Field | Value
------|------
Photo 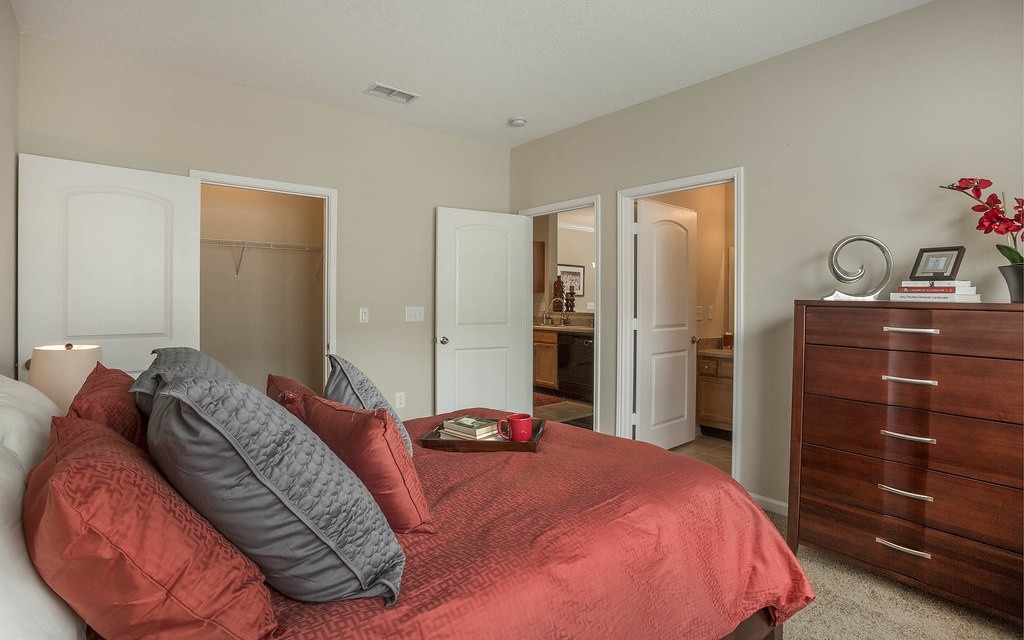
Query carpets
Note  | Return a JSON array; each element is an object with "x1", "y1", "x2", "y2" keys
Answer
[
  {"x1": 762, "y1": 509, "x2": 1024, "y2": 640},
  {"x1": 533, "y1": 392, "x2": 565, "y2": 407}
]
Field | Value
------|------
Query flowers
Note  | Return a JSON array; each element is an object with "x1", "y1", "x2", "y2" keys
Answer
[{"x1": 939, "y1": 178, "x2": 1024, "y2": 264}]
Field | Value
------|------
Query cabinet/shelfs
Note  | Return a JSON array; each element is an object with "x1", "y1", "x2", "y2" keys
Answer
[
  {"x1": 696, "y1": 355, "x2": 734, "y2": 432},
  {"x1": 786, "y1": 300, "x2": 1024, "y2": 628},
  {"x1": 533, "y1": 329, "x2": 570, "y2": 392}
]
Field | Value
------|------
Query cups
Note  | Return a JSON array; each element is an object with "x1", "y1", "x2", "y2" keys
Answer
[{"x1": 497, "y1": 413, "x2": 532, "y2": 442}]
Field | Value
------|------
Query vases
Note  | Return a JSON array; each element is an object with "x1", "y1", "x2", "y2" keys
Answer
[{"x1": 998, "y1": 265, "x2": 1024, "y2": 303}]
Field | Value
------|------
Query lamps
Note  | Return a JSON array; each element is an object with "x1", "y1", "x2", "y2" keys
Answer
[{"x1": 27, "y1": 343, "x2": 103, "y2": 415}]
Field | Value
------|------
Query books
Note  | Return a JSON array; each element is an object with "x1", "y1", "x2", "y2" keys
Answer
[
  {"x1": 437, "y1": 414, "x2": 506, "y2": 443},
  {"x1": 889, "y1": 280, "x2": 981, "y2": 303}
]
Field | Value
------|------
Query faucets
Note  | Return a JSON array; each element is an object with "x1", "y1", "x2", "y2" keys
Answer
[{"x1": 548, "y1": 298, "x2": 565, "y2": 318}]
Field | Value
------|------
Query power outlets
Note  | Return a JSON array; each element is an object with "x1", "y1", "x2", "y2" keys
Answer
[{"x1": 395, "y1": 392, "x2": 406, "y2": 408}]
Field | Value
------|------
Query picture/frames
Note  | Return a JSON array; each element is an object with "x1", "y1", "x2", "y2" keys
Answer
[
  {"x1": 557, "y1": 264, "x2": 585, "y2": 297},
  {"x1": 909, "y1": 246, "x2": 966, "y2": 280}
]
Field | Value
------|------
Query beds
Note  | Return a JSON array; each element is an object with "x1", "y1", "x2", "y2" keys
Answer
[{"x1": 1, "y1": 374, "x2": 815, "y2": 639}]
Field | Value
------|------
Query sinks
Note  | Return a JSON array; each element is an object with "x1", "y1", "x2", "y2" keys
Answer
[{"x1": 533, "y1": 323, "x2": 559, "y2": 330}]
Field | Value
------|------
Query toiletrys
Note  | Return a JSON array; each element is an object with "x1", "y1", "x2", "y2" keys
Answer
[
  {"x1": 546, "y1": 312, "x2": 552, "y2": 325},
  {"x1": 542, "y1": 310, "x2": 547, "y2": 325}
]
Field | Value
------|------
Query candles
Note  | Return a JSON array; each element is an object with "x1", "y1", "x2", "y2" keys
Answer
[
  {"x1": 570, "y1": 286, "x2": 574, "y2": 293},
  {"x1": 566, "y1": 292, "x2": 570, "y2": 299}
]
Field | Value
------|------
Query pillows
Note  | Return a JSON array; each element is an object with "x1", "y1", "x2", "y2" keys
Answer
[
  {"x1": 129, "y1": 347, "x2": 239, "y2": 417},
  {"x1": 23, "y1": 416, "x2": 279, "y2": 639},
  {"x1": 147, "y1": 373, "x2": 405, "y2": 607},
  {"x1": 324, "y1": 354, "x2": 413, "y2": 458},
  {"x1": 66, "y1": 361, "x2": 148, "y2": 454},
  {"x1": 267, "y1": 373, "x2": 437, "y2": 535}
]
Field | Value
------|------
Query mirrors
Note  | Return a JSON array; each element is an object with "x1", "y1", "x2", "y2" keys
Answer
[{"x1": 548, "y1": 207, "x2": 595, "y2": 315}]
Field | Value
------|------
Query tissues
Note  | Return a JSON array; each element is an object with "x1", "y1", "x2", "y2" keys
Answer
[{"x1": 722, "y1": 331, "x2": 734, "y2": 349}]
